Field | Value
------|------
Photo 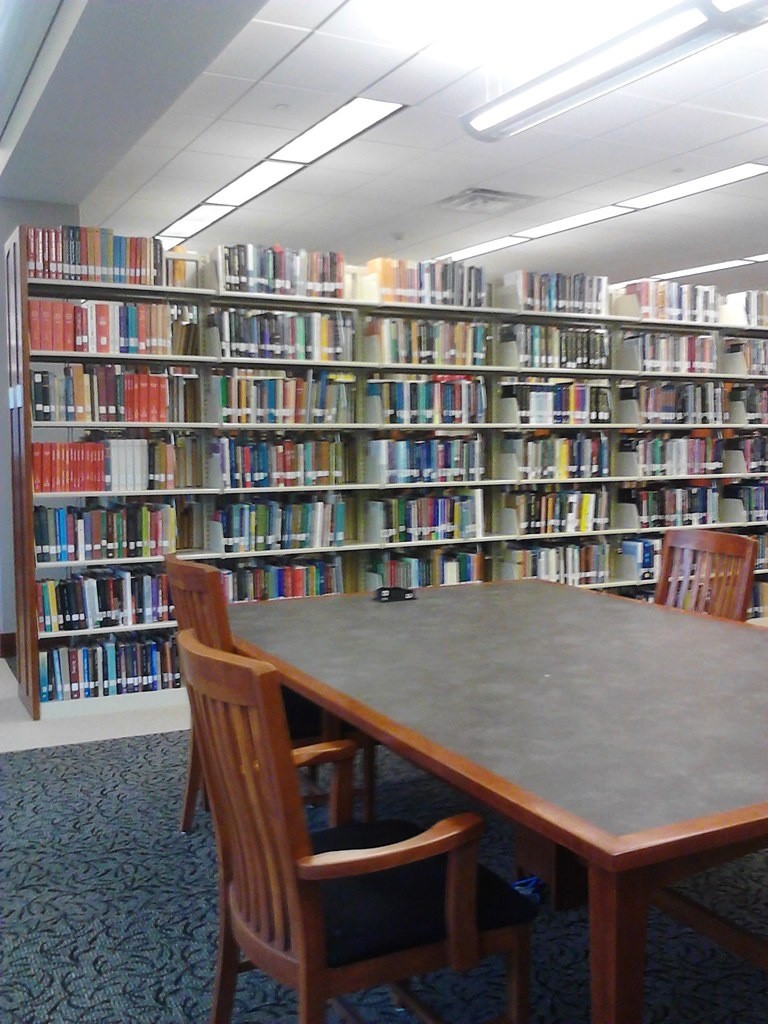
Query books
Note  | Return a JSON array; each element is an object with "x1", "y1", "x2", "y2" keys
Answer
[{"x1": 21, "y1": 222, "x2": 768, "y2": 705}]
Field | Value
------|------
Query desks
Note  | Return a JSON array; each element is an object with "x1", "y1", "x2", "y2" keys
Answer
[{"x1": 209, "y1": 575, "x2": 768, "y2": 1024}]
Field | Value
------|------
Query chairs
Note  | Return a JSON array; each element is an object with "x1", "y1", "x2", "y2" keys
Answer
[
  {"x1": 171, "y1": 627, "x2": 536, "y2": 1024},
  {"x1": 654, "y1": 530, "x2": 759, "y2": 624},
  {"x1": 162, "y1": 553, "x2": 376, "y2": 834}
]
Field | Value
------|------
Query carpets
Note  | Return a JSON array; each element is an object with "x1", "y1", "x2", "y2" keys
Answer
[{"x1": 0, "y1": 727, "x2": 767, "y2": 1024}]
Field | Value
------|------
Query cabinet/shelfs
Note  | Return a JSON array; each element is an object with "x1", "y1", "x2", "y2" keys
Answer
[{"x1": 1, "y1": 224, "x2": 768, "y2": 721}]
